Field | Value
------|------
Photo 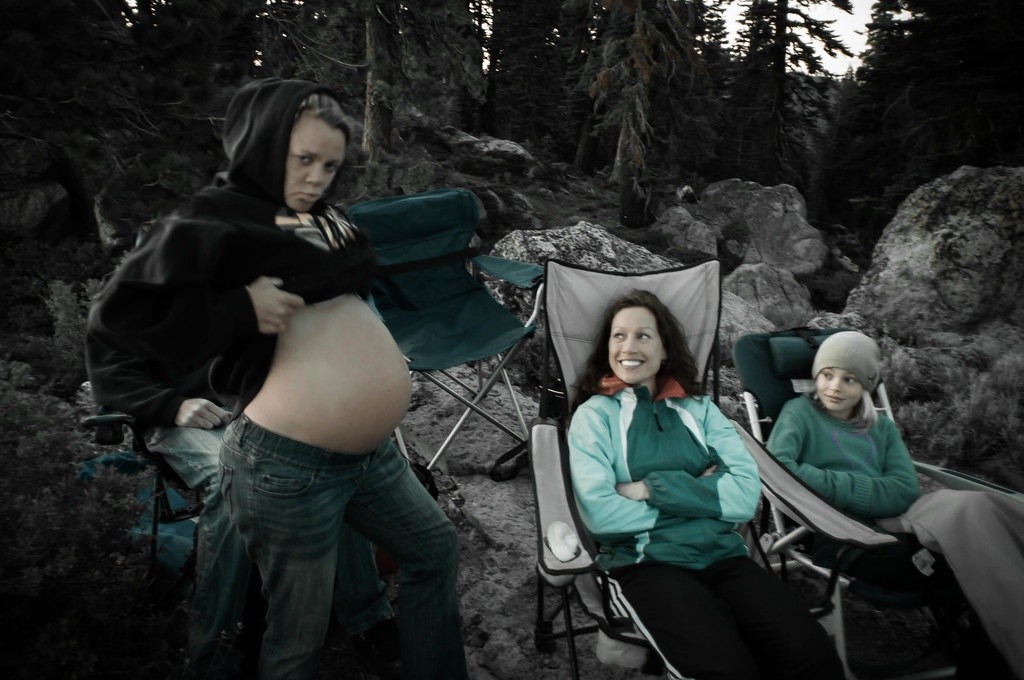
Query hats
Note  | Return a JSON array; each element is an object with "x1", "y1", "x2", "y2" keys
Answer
[{"x1": 812, "y1": 331, "x2": 880, "y2": 391}]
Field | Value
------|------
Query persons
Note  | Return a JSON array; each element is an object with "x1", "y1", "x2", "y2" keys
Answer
[
  {"x1": 79, "y1": 82, "x2": 412, "y2": 679},
  {"x1": 569, "y1": 289, "x2": 848, "y2": 680},
  {"x1": 767, "y1": 325, "x2": 1023, "y2": 679},
  {"x1": 145, "y1": 77, "x2": 467, "y2": 680}
]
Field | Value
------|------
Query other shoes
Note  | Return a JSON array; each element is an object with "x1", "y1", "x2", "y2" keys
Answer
[{"x1": 347, "y1": 617, "x2": 402, "y2": 678}]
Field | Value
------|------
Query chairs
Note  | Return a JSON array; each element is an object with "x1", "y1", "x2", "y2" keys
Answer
[
  {"x1": 82, "y1": 404, "x2": 204, "y2": 580},
  {"x1": 392, "y1": 253, "x2": 545, "y2": 476},
  {"x1": 528, "y1": 258, "x2": 1024, "y2": 680}
]
